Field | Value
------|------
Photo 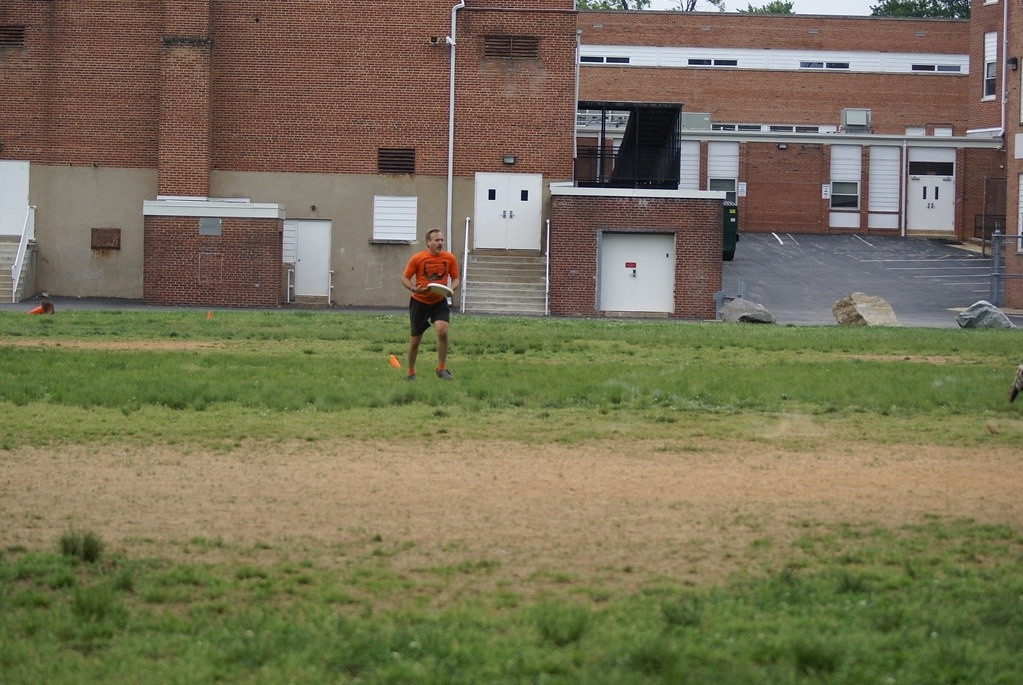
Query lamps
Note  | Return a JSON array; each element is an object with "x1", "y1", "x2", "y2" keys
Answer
[
  {"x1": 777, "y1": 143, "x2": 788, "y2": 149},
  {"x1": 1006, "y1": 57, "x2": 1017, "y2": 70},
  {"x1": 502, "y1": 156, "x2": 516, "y2": 164}
]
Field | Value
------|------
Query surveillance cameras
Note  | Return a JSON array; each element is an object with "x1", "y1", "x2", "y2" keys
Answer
[{"x1": 446, "y1": 36, "x2": 456, "y2": 46}]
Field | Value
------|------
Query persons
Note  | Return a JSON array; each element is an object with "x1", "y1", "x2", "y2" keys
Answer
[{"x1": 402, "y1": 229, "x2": 460, "y2": 382}]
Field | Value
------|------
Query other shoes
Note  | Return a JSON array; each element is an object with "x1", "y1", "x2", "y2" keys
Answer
[
  {"x1": 408, "y1": 374, "x2": 416, "y2": 382},
  {"x1": 436, "y1": 368, "x2": 452, "y2": 380}
]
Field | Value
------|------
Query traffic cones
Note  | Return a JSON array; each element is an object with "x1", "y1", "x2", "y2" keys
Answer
[
  {"x1": 207, "y1": 310, "x2": 212, "y2": 319},
  {"x1": 23, "y1": 302, "x2": 54, "y2": 314},
  {"x1": 389, "y1": 355, "x2": 403, "y2": 370}
]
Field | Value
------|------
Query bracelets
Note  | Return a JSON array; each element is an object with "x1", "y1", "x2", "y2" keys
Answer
[{"x1": 412, "y1": 288, "x2": 414, "y2": 292}]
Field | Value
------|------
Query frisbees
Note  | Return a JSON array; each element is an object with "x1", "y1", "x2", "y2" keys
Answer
[{"x1": 427, "y1": 282, "x2": 452, "y2": 296}]
